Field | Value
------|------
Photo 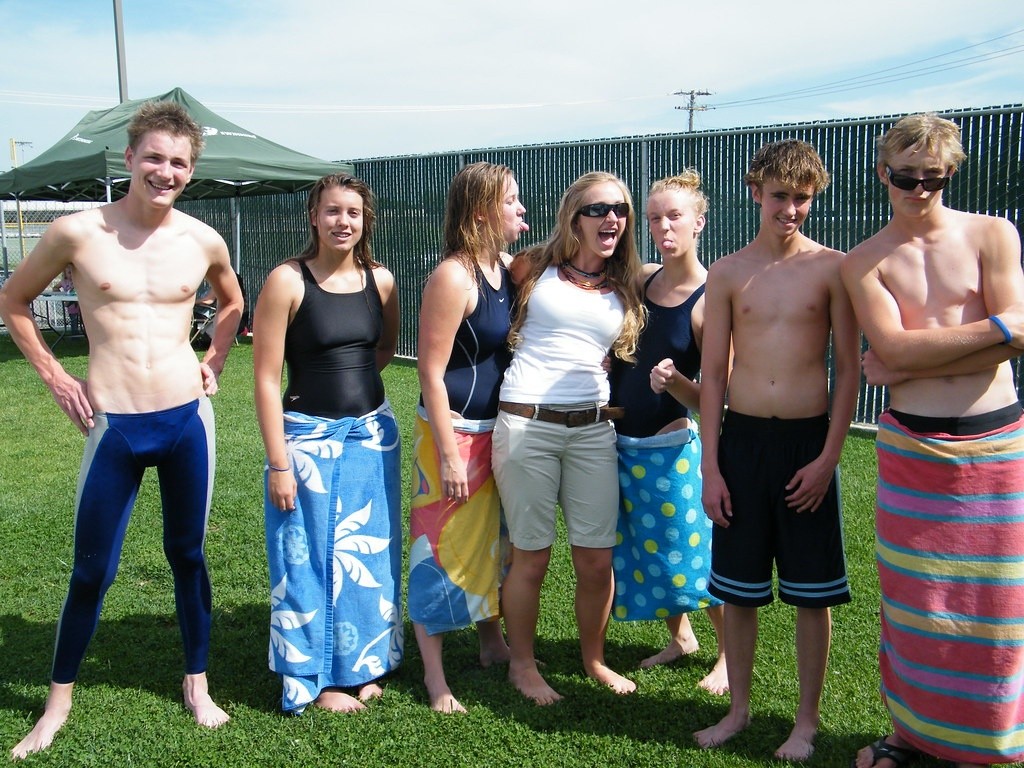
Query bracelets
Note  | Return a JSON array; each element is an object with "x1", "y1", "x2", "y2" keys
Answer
[
  {"x1": 268, "y1": 463, "x2": 290, "y2": 472},
  {"x1": 985, "y1": 315, "x2": 1014, "y2": 343}
]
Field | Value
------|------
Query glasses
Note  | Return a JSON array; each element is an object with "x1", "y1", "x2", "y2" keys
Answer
[
  {"x1": 885, "y1": 163, "x2": 950, "y2": 191},
  {"x1": 576, "y1": 203, "x2": 629, "y2": 218}
]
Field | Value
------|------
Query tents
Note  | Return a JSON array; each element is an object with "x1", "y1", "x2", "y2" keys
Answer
[{"x1": 0, "y1": 85, "x2": 356, "y2": 351}]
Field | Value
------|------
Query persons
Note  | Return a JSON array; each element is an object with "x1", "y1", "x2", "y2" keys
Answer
[
  {"x1": 0, "y1": 98, "x2": 245, "y2": 763},
  {"x1": 409, "y1": 161, "x2": 530, "y2": 714},
  {"x1": 691, "y1": 138, "x2": 862, "y2": 760},
  {"x1": 608, "y1": 165, "x2": 735, "y2": 697},
  {"x1": 52, "y1": 263, "x2": 86, "y2": 336},
  {"x1": 840, "y1": 113, "x2": 1024, "y2": 768},
  {"x1": 192, "y1": 280, "x2": 220, "y2": 339},
  {"x1": 252, "y1": 173, "x2": 404, "y2": 714},
  {"x1": 491, "y1": 171, "x2": 656, "y2": 706}
]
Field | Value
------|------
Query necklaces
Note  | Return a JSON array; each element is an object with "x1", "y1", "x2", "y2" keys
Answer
[{"x1": 557, "y1": 259, "x2": 621, "y2": 292}]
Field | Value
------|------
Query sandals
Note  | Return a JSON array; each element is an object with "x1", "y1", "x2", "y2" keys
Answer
[{"x1": 849, "y1": 735, "x2": 918, "y2": 768}]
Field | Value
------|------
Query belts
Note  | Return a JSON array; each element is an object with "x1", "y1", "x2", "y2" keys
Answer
[{"x1": 499, "y1": 401, "x2": 626, "y2": 428}]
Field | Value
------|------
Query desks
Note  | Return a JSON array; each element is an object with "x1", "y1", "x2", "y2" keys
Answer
[{"x1": 31, "y1": 289, "x2": 88, "y2": 352}]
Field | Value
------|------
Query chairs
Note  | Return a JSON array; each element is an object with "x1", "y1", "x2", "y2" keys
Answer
[{"x1": 189, "y1": 303, "x2": 239, "y2": 346}]
